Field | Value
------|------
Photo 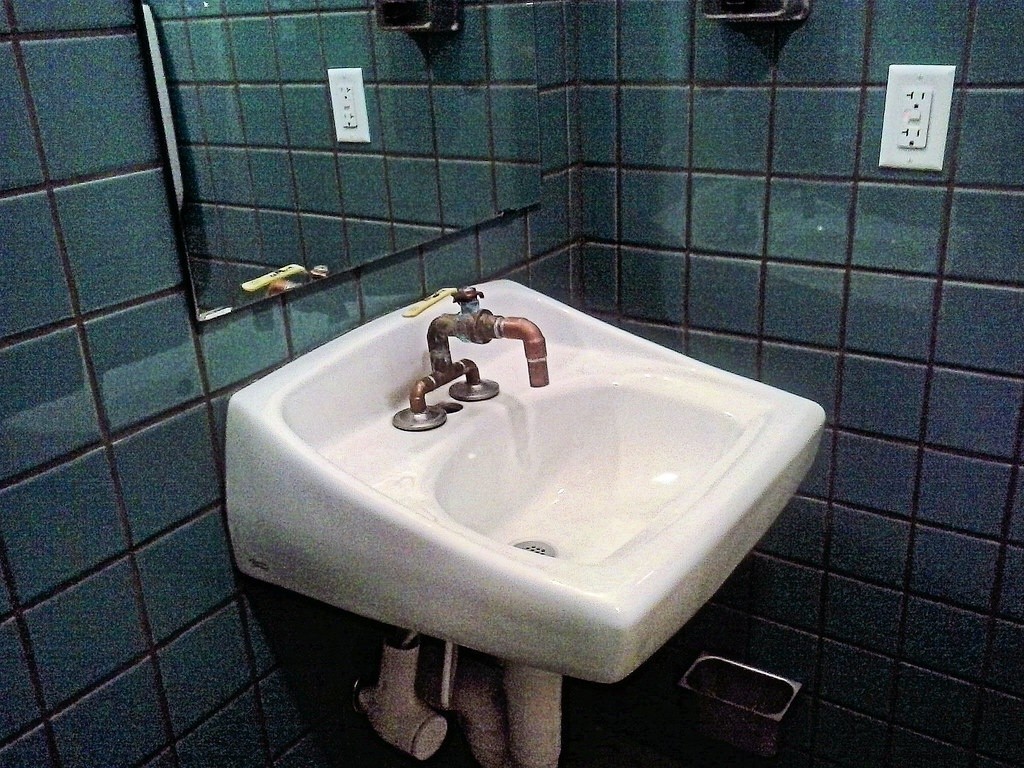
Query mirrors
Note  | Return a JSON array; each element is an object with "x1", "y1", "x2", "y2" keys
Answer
[{"x1": 138, "y1": 0, "x2": 544, "y2": 323}]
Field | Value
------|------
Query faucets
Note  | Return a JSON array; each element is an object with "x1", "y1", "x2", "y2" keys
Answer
[
  {"x1": 400, "y1": 285, "x2": 550, "y2": 388},
  {"x1": 241, "y1": 262, "x2": 329, "y2": 300}
]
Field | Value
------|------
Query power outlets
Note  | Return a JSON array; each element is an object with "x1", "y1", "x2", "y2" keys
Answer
[
  {"x1": 325, "y1": 68, "x2": 371, "y2": 143},
  {"x1": 876, "y1": 64, "x2": 956, "y2": 173}
]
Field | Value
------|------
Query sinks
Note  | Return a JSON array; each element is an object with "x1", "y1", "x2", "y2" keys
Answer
[{"x1": 223, "y1": 278, "x2": 827, "y2": 686}]
